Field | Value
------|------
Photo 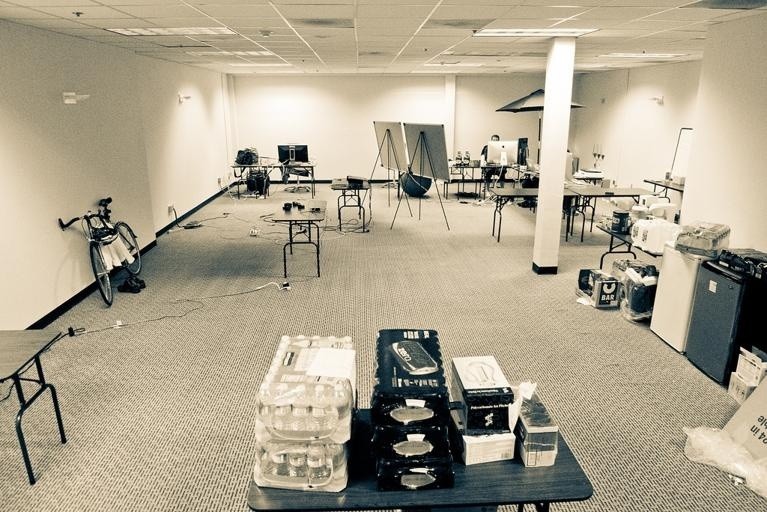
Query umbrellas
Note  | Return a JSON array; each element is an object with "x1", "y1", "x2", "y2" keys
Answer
[{"x1": 495, "y1": 88, "x2": 586, "y2": 165}]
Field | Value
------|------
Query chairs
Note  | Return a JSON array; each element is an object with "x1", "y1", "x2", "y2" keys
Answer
[{"x1": 285, "y1": 168, "x2": 311, "y2": 193}]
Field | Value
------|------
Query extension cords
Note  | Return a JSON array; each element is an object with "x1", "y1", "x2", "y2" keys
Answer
[
  {"x1": 475, "y1": 199, "x2": 484, "y2": 205},
  {"x1": 283, "y1": 282, "x2": 291, "y2": 291},
  {"x1": 249, "y1": 227, "x2": 258, "y2": 236}
]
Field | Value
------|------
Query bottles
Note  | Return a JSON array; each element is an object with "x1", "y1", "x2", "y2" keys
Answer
[
  {"x1": 253, "y1": 334, "x2": 355, "y2": 492},
  {"x1": 456, "y1": 151, "x2": 462, "y2": 164},
  {"x1": 464, "y1": 151, "x2": 470, "y2": 166}
]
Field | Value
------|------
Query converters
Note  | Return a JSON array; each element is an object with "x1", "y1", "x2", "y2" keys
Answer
[{"x1": 283, "y1": 283, "x2": 290, "y2": 287}]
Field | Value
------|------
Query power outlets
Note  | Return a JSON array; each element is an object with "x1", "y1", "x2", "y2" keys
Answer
[{"x1": 169, "y1": 203, "x2": 174, "y2": 212}]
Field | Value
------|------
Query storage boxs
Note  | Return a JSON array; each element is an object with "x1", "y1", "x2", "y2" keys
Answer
[
  {"x1": 728, "y1": 372, "x2": 757, "y2": 404},
  {"x1": 373, "y1": 452, "x2": 457, "y2": 494},
  {"x1": 449, "y1": 402, "x2": 516, "y2": 466},
  {"x1": 365, "y1": 327, "x2": 452, "y2": 430},
  {"x1": 366, "y1": 387, "x2": 450, "y2": 466},
  {"x1": 736, "y1": 346, "x2": 767, "y2": 385},
  {"x1": 451, "y1": 356, "x2": 514, "y2": 434},
  {"x1": 515, "y1": 388, "x2": 559, "y2": 467}
]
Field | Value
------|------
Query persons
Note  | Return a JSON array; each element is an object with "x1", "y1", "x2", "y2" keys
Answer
[{"x1": 480, "y1": 135, "x2": 507, "y2": 191}]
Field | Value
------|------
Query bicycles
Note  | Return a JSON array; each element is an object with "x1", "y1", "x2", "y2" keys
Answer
[{"x1": 56, "y1": 193, "x2": 144, "y2": 306}]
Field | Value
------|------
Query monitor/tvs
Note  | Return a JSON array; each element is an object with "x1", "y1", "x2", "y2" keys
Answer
[
  {"x1": 278, "y1": 145, "x2": 308, "y2": 165},
  {"x1": 517, "y1": 138, "x2": 529, "y2": 165},
  {"x1": 487, "y1": 141, "x2": 518, "y2": 166}
]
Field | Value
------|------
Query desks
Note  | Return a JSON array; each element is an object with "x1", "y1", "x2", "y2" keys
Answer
[
  {"x1": 442, "y1": 152, "x2": 685, "y2": 270},
  {"x1": 272, "y1": 200, "x2": 329, "y2": 278},
  {"x1": 0, "y1": 329, "x2": 67, "y2": 486},
  {"x1": 330, "y1": 176, "x2": 372, "y2": 232},
  {"x1": 231, "y1": 161, "x2": 319, "y2": 199},
  {"x1": 247, "y1": 388, "x2": 593, "y2": 511}
]
